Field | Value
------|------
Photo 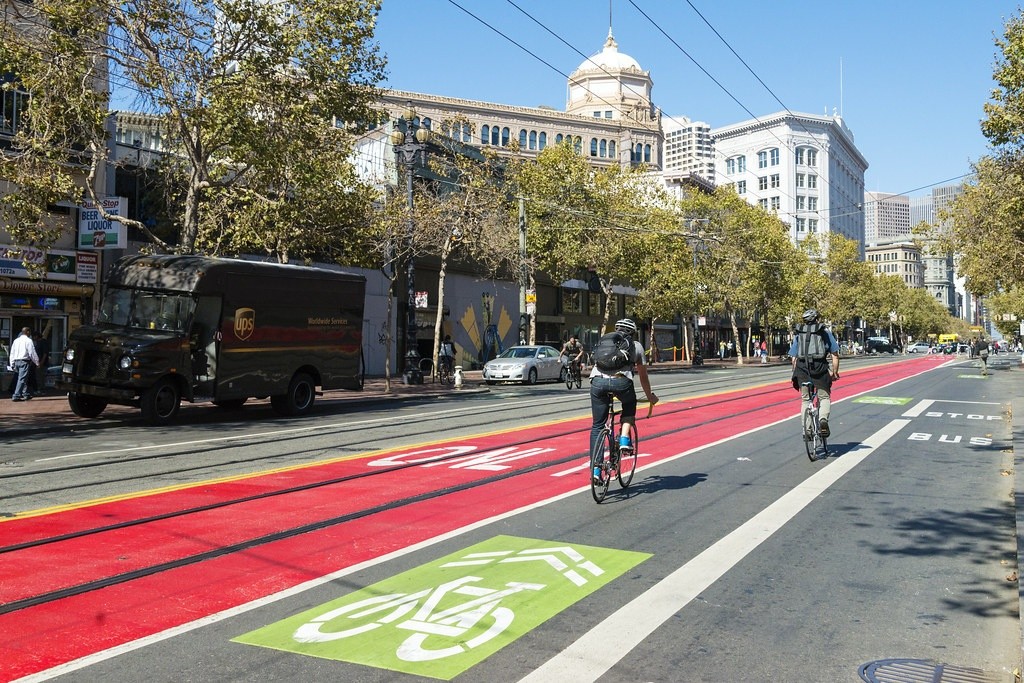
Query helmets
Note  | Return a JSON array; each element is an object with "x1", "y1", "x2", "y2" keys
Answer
[
  {"x1": 615, "y1": 317, "x2": 638, "y2": 332},
  {"x1": 802, "y1": 309, "x2": 818, "y2": 324}
]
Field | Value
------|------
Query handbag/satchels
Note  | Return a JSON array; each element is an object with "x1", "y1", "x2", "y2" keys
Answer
[
  {"x1": 446, "y1": 355, "x2": 454, "y2": 362},
  {"x1": 979, "y1": 349, "x2": 988, "y2": 358}
]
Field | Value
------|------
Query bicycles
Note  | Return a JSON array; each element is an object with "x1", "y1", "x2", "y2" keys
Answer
[
  {"x1": 558, "y1": 358, "x2": 584, "y2": 390},
  {"x1": 436, "y1": 352, "x2": 458, "y2": 386},
  {"x1": 799, "y1": 376, "x2": 840, "y2": 461},
  {"x1": 588, "y1": 392, "x2": 659, "y2": 503}
]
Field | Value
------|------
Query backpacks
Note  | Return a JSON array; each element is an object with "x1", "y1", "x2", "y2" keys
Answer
[
  {"x1": 797, "y1": 322, "x2": 828, "y2": 359},
  {"x1": 596, "y1": 331, "x2": 636, "y2": 371}
]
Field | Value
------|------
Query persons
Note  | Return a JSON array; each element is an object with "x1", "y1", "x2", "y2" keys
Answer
[
  {"x1": 1018, "y1": 341, "x2": 1023, "y2": 354},
  {"x1": 927, "y1": 341, "x2": 932, "y2": 354},
  {"x1": 588, "y1": 318, "x2": 659, "y2": 483},
  {"x1": 726, "y1": 340, "x2": 733, "y2": 358},
  {"x1": 848, "y1": 340, "x2": 860, "y2": 355},
  {"x1": 753, "y1": 339, "x2": 761, "y2": 357},
  {"x1": 9, "y1": 326, "x2": 49, "y2": 401},
  {"x1": 787, "y1": 309, "x2": 840, "y2": 440},
  {"x1": 720, "y1": 340, "x2": 726, "y2": 358},
  {"x1": 978, "y1": 336, "x2": 990, "y2": 374},
  {"x1": 560, "y1": 335, "x2": 584, "y2": 386},
  {"x1": 761, "y1": 339, "x2": 768, "y2": 364},
  {"x1": 991, "y1": 340, "x2": 1000, "y2": 354},
  {"x1": 438, "y1": 335, "x2": 457, "y2": 373}
]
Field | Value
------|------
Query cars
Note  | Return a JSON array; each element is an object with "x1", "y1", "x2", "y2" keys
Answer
[
  {"x1": 482, "y1": 344, "x2": 571, "y2": 387},
  {"x1": 839, "y1": 332, "x2": 1024, "y2": 355}
]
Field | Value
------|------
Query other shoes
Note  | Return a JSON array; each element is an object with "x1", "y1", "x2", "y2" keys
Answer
[
  {"x1": 802, "y1": 433, "x2": 812, "y2": 441},
  {"x1": 982, "y1": 371, "x2": 987, "y2": 375},
  {"x1": 575, "y1": 376, "x2": 581, "y2": 385},
  {"x1": 24, "y1": 396, "x2": 33, "y2": 400},
  {"x1": 819, "y1": 419, "x2": 830, "y2": 437},
  {"x1": 12, "y1": 397, "x2": 25, "y2": 402}
]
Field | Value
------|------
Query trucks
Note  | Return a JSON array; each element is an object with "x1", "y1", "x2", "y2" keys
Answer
[{"x1": 56, "y1": 253, "x2": 367, "y2": 427}]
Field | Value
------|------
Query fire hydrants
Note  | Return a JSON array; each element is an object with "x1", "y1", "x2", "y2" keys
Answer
[{"x1": 452, "y1": 364, "x2": 466, "y2": 390}]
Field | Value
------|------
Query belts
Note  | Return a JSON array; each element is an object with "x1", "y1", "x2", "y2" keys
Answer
[{"x1": 14, "y1": 360, "x2": 31, "y2": 362}]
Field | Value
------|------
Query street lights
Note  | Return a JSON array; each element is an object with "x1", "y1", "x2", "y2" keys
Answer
[
  {"x1": 688, "y1": 218, "x2": 708, "y2": 366},
  {"x1": 389, "y1": 98, "x2": 432, "y2": 387}
]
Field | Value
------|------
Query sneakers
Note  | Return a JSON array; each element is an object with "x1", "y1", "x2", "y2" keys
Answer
[
  {"x1": 619, "y1": 436, "x2": 634, "y2": 451},
  {"x1": 593, "y1": 466, "x2": 601, "y2": 480}
]
